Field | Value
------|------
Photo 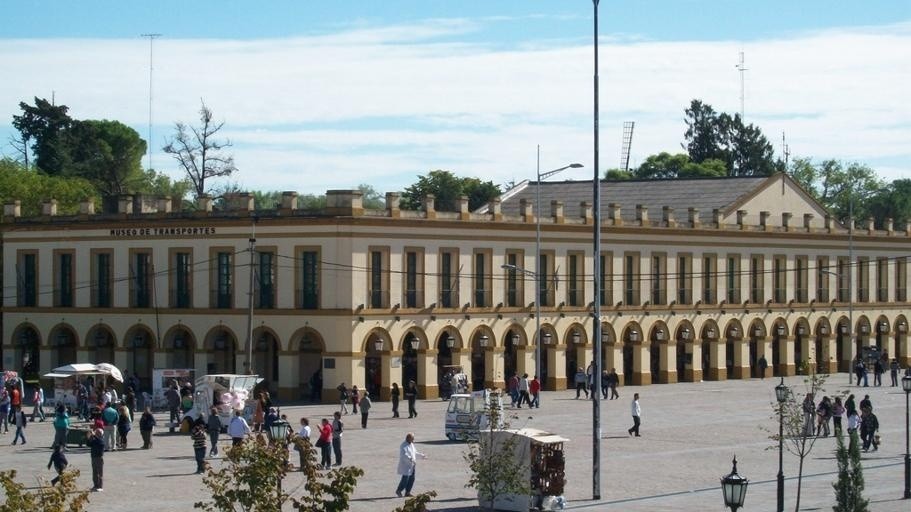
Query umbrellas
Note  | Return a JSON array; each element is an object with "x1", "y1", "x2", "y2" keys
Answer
[{"x1": 93, "y1": 362, "x2": 125, "y2": 392}]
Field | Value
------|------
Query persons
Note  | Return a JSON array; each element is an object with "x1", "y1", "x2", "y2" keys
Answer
[
  {"x1": 438, "y1": 368, "x2": 470, "y2": 402},
  {"x1": 395, "y1": 431, "x2": 427, "y2": 498},
  {"x1": 164, "y1": 379, "x2": 194, "y2": 423},
  {"x1": 390, "y1": 383, "x2": 399, "y2": 418},
  {"x1": 855, "y1": 346, "x2": 911, "y2": 387},
  {"x1": 253, "y1": 391, "x2": 277, "y2": 431},
  {"x1": 88, "y1": 428, "x2": 107, "y2": 493},
  {"x1": 759, "y1": 354, "x2": 768, "y2": 380},
  {"x1": 49, "y1": 442, "x2": 69, "y2": 487},
  {"x1": 337, "y1": 383, "x2": 360, "y2": 415},
  {"x1": 405, "y1": 381, "x2": 417, "y2": 418},
  {"x1": 227, "y1": 411, "x2": 251, "y2": 446},
  {"x1": 54, "y1": 385, "x2": 156, "y2": 451},
  {"x1": 297, "y1": 411, "x2": 343, "y2": 471},
  {"x1": 510, "y1": 371, "x2": 543, "y2": 409},
  {"x1": 1, "y1": 383, "x2": 45, "y2": 445},
  {"x1": 192, "y1": 425, "x2": 207, "y2": 474},
  {"x1": 627, "y1": 391, "x2": 644, "y2": 437},
  {"x1": 575, "y1": 361, "x2": 621, "y2": 401},
  {"x1": 360, "y1": 391, "x2": 371, "y2": 428},
  {"x1": 803, "y1": 392, "x2": 880, "y2": 453},
  {"x1": 195, "y1": 408, "x2": 221, "y2": 457}
]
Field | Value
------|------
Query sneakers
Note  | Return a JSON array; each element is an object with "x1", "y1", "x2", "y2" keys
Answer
[
  {"x1": 104, "y1": 447, "x2": 127, "y2": 451},
  {"x1": 11, "y1": 442, "x2": 16, "y2": 445},
  {"x1": 96, "y1": 488, "x2": 103, "y2": 492},
  {"x1": 396, "y1": 490, "x2": 401, "y2": 496},
  {"x1": 21, "y1": 441, "x2": 26, "y2": 445},
  {"x1": 89, "y1": 486, "x2": 96, "y2": 490},
  {"x1": 405, "y1": 494, "x2": 413, "y2": 496}
]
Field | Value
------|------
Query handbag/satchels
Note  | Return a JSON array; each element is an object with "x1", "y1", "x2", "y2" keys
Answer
[{"x1": 315, "y1": 438, "x2": 324, "y2": 447}]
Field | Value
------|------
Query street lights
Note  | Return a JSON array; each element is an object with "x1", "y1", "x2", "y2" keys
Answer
[
  {"x1": 268, "y1": 405, "x2": 291, "y2": 511},
  {"x1": 719, "y1": 451, "x2": 749, "y2": 511},
  {"x1": 772, "y1": 374, "x2": 790, "y2": 511},
  {"x1": 246, "y1": 236, "x2": 257, "y2": 373},
  {"x1": 900, "y1": 366, "x2": 910, "y2": 498},
  {"x1": 500, "y1": 159, "x2": 585, "y2": 406},
  {"x1": 819, "y1": 187, "x2": 894, "y2": 384}
]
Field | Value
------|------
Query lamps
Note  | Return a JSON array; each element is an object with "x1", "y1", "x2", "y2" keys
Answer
[
  {"x1": 820, "y1": 324, "x2": 828, "y2": 336},
  {"x1": 410, "y1": 321, "x2": 420, "y2": 350},
  {"x1": 602, "y1": 331, "x2": 609, "y2": 342},
  {"x1": 375, "y1": 323, "x2": 384, "y2": 351},
  {"x1": 512, "y1": 335, "x2": 521, "y2": 345},
  {"x1": 730, "y1": 326, "x2": 738, "y2": 336},
  {"x1": 798, "y1": 325, "x2": 806, "y2": 336},
  {"x1": 630, "y1": 330, "x2": 638, "y2": 341},
  {"x1": 880, "y1": 323, "x2": 888, "y2": 332},
  {"x1": 778, "y1": 325, "x2": 785, "y2": 336},
  {"x1": 899, "y1": 321, "x2": 907, "y2": 332},
  {"x1": 657, "y1": 329, "x2": 664, "y2": 341},
  {"x1": 708, "y1": 327, "x2": 715, "y2": 338},
  {"x1": 861, "y1": 323, "x2": 868, "y2": 332},
  {"x1": 680, "y1": 327, "x2": 690, "y2": 338},
  {"x1": 479, "y1": 335, "x2": 489, "y2": 346},
  {"x1": 840, "y1": 323, "x2": 848, "y2": 335},
  {"x1": 573, "y1": 332, "x2": 580, "y2": 343},
  {"x1": 543, "y1": 333, "x2": 552, "y2": 344},
  {"x1": 755, "y1": 326, "x2": 762, "y2": 336},
  {"x1": 447, "y1": 322, "x2": 455, "y2": 348}
]
